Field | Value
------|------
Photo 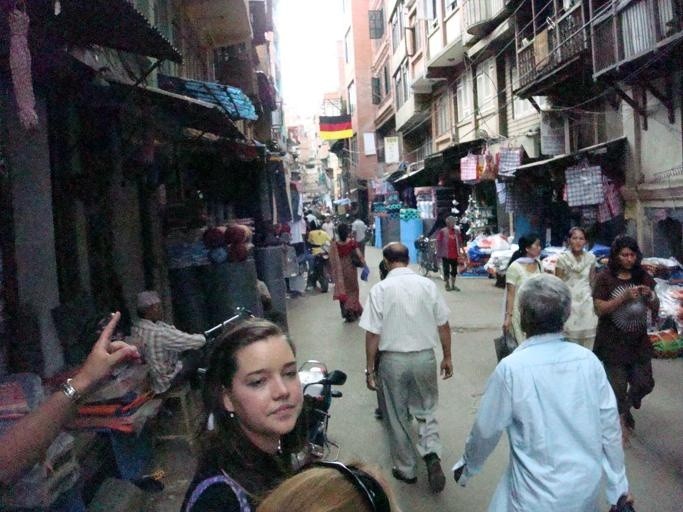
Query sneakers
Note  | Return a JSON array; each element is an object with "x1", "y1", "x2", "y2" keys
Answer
[
  {"x1": 445, "y1": 283, "x2": 460, "y2": 292},
  {"x1": 422, "y1": 452, "x2": 445, "y2": 493},
  {"x1": 392, "y1": 466, "x2": 417, "y2": 483}
]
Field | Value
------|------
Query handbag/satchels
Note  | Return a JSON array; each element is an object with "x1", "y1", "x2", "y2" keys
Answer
[
  {"x1": 351, "y1": 246, "x2": 362, "y2": 266},
  {"x1": 460, "y1": 145, "x2": 523, "y2": 185},
  {"x1": 562, "y1": 157, "x2": 624, "y2": 225}
]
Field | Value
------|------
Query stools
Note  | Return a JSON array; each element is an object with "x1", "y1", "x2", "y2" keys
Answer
[{"x1": 154, "y1": 382, "x2": 202, "y2": 456}]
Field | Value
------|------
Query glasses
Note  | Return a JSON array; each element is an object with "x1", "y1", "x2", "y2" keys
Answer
[{"x1": 293, "y1": 459, "x2": 390, "y2": 512}]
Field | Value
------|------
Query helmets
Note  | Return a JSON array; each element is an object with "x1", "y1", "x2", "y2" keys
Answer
[{"x1": 310, "y1": 220, "x2": 322, "y2": 230}]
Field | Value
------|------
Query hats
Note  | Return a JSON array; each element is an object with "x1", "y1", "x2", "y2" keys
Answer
[
  {"x1": 609, "y1": 282, "x2": 657, "y2": 335},
  {"x1": 134, "y1": 291, "x2": 162, "y2": 310}
]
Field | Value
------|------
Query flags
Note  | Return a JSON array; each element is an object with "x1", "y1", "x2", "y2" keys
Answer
[{"x1": 319, "y1": 115, "x2": 353, "y2": 140}]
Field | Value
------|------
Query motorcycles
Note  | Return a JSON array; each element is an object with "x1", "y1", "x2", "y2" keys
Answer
[
  {"x1": 306, "y1": 251, "x2": 330, "y2": 294},
  {"x1": 189, "y1": 359, "x2": 348, "y2": 475}
]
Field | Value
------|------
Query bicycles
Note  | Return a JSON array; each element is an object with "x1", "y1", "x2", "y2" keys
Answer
[{"x1": 412, "y1": 237, "x2": 444, "y2": 280}]
